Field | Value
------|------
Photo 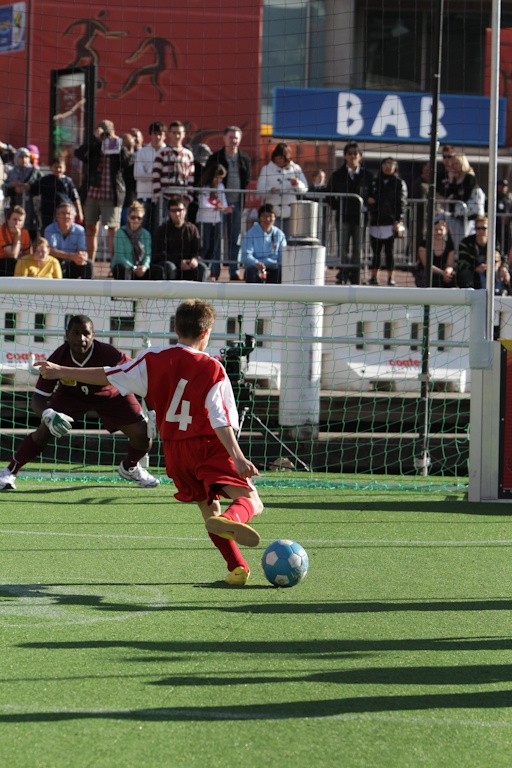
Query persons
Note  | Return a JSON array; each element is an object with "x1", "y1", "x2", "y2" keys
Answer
[
  {"x1": 457, "y1": 215, "x2": 511, "y2": 290},
  {"x1": 256, "y1": 142, "x2": 309, "y2": 239},
  {"x1": 207, "y1": 126, "x2": 252, "y2": 280},
  {"x1": 368, "y1": 158, "x2": 408, "y2": 285},
  {"x1": 304, "y1": 169, "x2": 329, "y2": 203},
  {"x1": 0, "y1": 315, "x2": 160, "y2": 489},
  {"x1": 241, "y1": 203, "x2": 287, "y2": 284},
  {"x1": 44, "y1": 201, "x2": 93, "y2": 279},
  {"x1": 110, "y1": 199, "x2": 153, "y2": 280},
  {"x1": 413, "y1": 219, "x2": 458, "y2": 287},
  {"x1": 32, "y1": 297, "x2": 264, "y2": 585},
  {"x1": 152, "y1": 195, "x2": 206, "y2": 282},
  {"x1": 0, "y1": 119, "x2": 512, "y2": 296}
]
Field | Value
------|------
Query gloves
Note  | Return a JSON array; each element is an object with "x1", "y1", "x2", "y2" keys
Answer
[{"x1": 42, "y1": 409, "x2": 74, "y2": 438}]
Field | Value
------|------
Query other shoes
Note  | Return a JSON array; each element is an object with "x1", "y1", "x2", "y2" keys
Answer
[
  {"x1": 363, "y1": 278, "x2": 378, "y2": 285},
  {"x1": 387, "y1": 278, "x2": 395, "y2": 284}
]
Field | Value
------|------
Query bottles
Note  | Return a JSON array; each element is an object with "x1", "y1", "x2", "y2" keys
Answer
[{"x1": 259, "y1": 269, "x2": 266, "y2": 283}]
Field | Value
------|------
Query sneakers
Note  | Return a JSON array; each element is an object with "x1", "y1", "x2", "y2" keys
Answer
[
  {"x1": 0, "y1": 468, "x2": 16, "y2": 490},
  {"x1": 119, "y1": 460, "x2": 160, "y2": 486},
  {"x1": 226, "y1": 565, "x2": 249, "y2": 586},
  {"x1": 206, "y1": 515, "x2": 261, "y2": 547}
]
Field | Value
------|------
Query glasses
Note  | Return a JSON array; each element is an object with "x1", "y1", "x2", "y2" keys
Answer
[
  {"x1": 475, "y1": 226, "x2": 487, "y2": 231},
  {"x1": 170, "y1": 208, "x2": 184, "y2": 212},
  {"x1": 443, "y1": 155, "x2": 453, "y2": 160},
  {"x1": 130, "y1": 216, "x2": 143, "y2": 220}
]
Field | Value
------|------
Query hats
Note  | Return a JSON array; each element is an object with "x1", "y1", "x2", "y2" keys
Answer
[{"x1": 28, "y1": 144, "x2": 39, "y2": 155}]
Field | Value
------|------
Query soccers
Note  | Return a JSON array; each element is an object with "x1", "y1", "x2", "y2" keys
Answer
[{"x1": 261, "y1": 540, "x2": 310, "y2": 587}]
